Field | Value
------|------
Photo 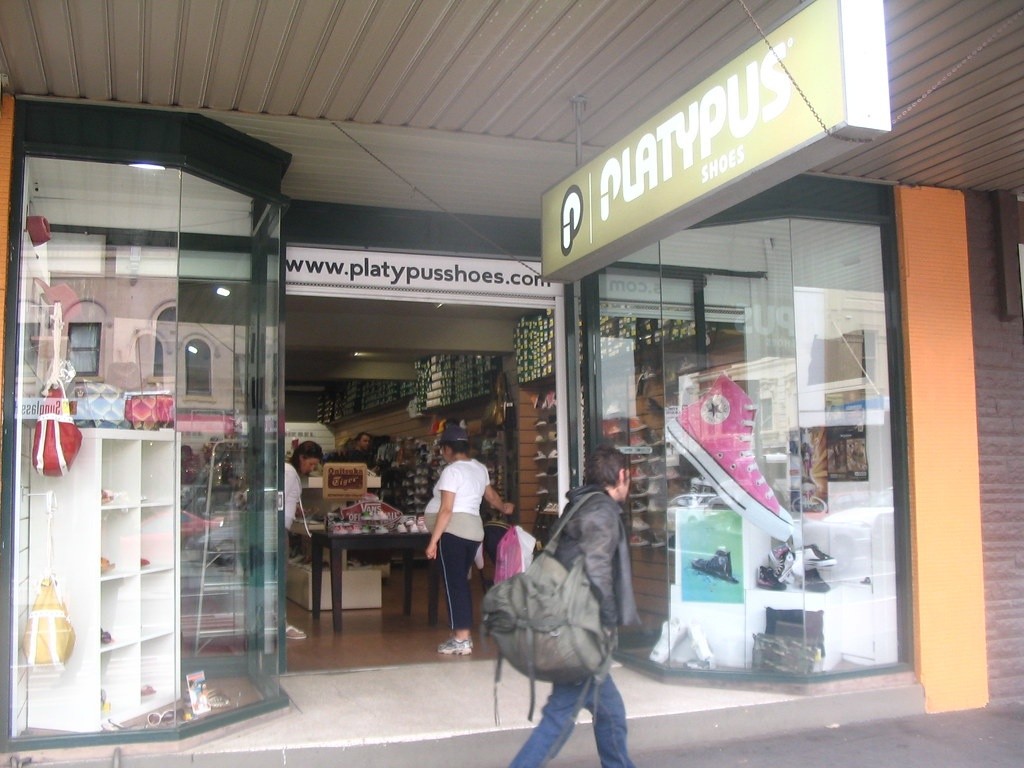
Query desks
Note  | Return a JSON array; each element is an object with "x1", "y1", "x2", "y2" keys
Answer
[{"x1": 309, "y1": 529, "x2": 442, "y2": 633}]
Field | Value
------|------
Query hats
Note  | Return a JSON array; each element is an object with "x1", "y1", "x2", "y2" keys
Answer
[{"x1": 436, "y1": 425, "x2": 471, "y2": 444}]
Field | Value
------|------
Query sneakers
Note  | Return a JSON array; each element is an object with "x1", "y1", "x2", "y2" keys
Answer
[
  {"x1": 438, "y1": 639, "x2": 474, "y2": 656},
  {"x1": 329, "y1": 434, "x2": 505, "y2": 534},
  {"x1": 522, "y1": 369, "x2": 666, "y2": 563},
  {"x1": 666, "y1": 371, "x2": 840, "y2": 591}
]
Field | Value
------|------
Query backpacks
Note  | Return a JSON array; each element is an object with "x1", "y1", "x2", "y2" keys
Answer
[
  {"x1": 33, "y1": 374, "x2": 83, "y2": 478},
  {"x1": 17, "y1": 572, "x2": 74, "y2": 664},
  {"x1": 476, "y1": 489, "x2": 618, "y2": 686}
]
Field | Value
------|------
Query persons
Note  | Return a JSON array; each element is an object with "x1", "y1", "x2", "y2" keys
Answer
[
  {"x1": 284, "y1": 441, "x2": 323, "y2": 644},
  {"x1": 507, "y1": 447, "x2": 641, "y2": 767},
  {"x1": 423, "y1": 426, "x2": 514, "y2": 656},
  {"x1": 349, "y1": 430, "x2": 373, "y2": 459}
]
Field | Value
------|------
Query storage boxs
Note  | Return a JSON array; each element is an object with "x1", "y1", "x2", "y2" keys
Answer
[
  {"x1": 316, "y1": 379, "x2": 415, "y2": 425},
  {"x1": 415, "y1": 303, "x2": 717, "y2": 414}
]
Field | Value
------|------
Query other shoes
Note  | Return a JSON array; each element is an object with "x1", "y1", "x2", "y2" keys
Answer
[{"x1": 284, "y1": 626, "x2": 309, "y2": 640}]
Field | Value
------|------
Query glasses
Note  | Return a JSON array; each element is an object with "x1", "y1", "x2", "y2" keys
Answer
[{"x1": 149, "y1": 706, "x2": 186, "y2": 726}]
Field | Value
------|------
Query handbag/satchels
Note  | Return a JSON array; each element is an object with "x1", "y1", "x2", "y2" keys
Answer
[
  {"x1": 648, "y1": 614, "x2": 717, "y2": 670},
  {"x1": 33, "y1": 277, "x2": 176, "y2": 430},
  {"x1": 753, "y1": 605, "x2": 827, "y2": 676}
]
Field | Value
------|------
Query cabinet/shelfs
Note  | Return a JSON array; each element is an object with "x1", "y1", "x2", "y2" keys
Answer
[
  {"x1": 677, "y1": 356, "x2": 797, "y2": 501},
  {"x1": 187, "y1": 439, "x2": 250, "y2": 658},
  {"x1": 27, "y1": 426, "x2": 185, "y2": 732},
  {"x1": 286, "y1": 475, "x2": 386, "y2": 612},
  {"x1": 666, "y1": 503, "x2": 897, "y2": 673}
]
